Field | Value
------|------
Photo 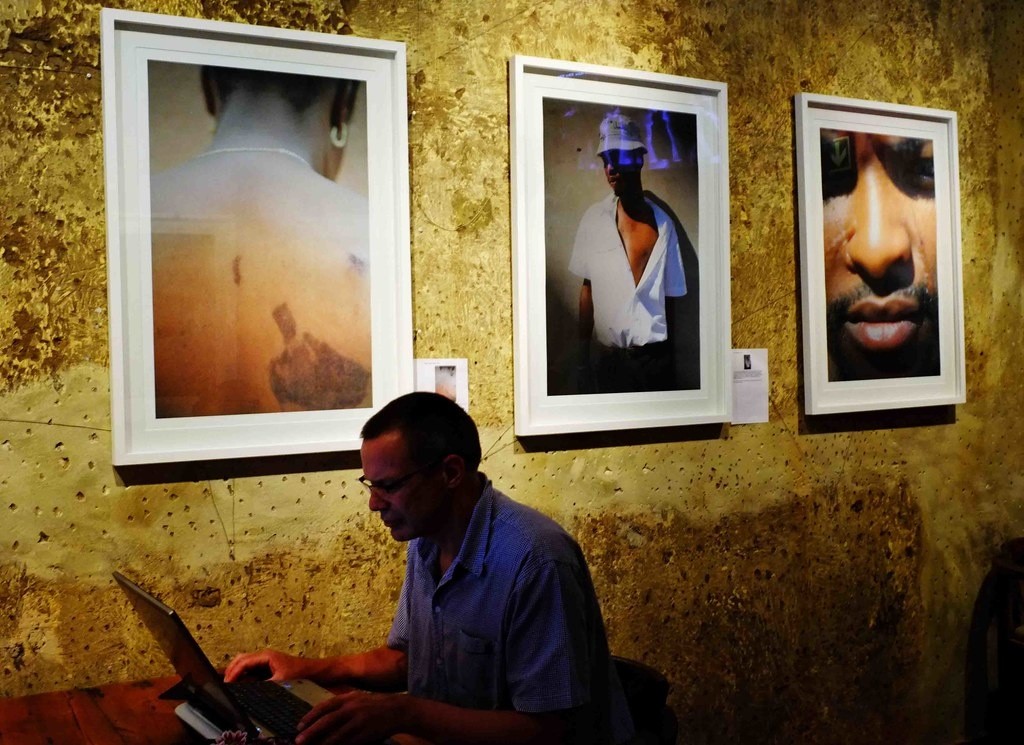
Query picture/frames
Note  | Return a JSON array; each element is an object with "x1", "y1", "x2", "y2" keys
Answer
[
  {"x1": 100, "y1": 6, "x2": 414, "y2": 466},
  {"x1": 796, "y1": 92, "x2": 966, "y2": 415},
  {"x1": 505, "y1": 55, "x2": 733, "y2": 438}
]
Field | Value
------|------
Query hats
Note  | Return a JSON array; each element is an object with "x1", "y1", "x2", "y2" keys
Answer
[{"x1": 597, "y1": 114, "x2": 648, "y2": 156}]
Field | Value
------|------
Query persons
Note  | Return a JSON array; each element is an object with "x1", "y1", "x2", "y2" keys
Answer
[
  {"x1": 224, "y1": 395, "x2": 633, "y2": 742},
  {"x1": 818, "y1": 127, "x2": 942, "y2": 382},
  {"x1": 568, "y1": 114, "x2": 688, "y2": 391},
  {"x1": 150, "y1": 62, "x2": 376, "y2": 419}
]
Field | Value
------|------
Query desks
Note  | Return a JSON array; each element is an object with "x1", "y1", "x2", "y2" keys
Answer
[{"x1": 0, "y1": 668, "x2": 436, "y2": 745}]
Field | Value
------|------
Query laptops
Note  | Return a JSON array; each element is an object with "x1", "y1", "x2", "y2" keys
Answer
[{"x1": 111, "y1": 571, "x2": 327, "y2": 745}]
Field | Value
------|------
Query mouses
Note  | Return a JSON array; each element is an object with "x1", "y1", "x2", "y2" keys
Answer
[{"x1": 236, "y1": 666, "x2": 272, "y2": 682}]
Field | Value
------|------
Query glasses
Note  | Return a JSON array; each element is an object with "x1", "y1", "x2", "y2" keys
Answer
[{"x1": 359, "y1": 458, "x2": 441, "y2": 498}]
